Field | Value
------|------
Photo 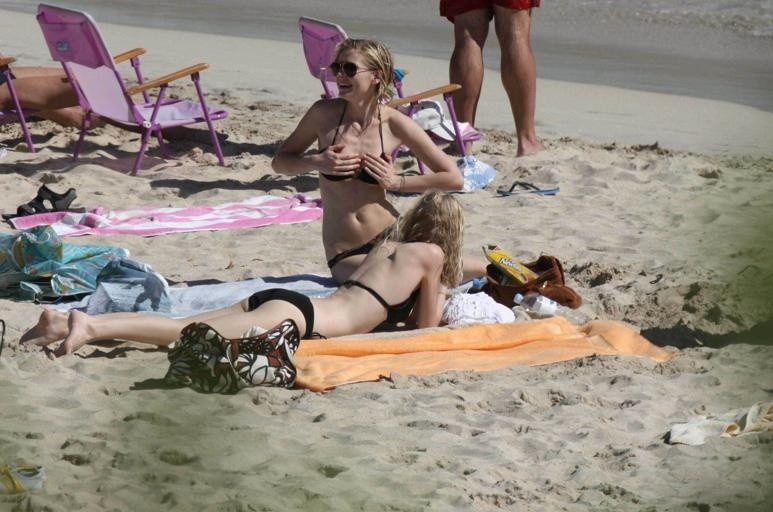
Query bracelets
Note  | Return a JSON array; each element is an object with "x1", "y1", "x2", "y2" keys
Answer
[{"x1": 397, "y1": 176, "x2": 406, "y2": 196}]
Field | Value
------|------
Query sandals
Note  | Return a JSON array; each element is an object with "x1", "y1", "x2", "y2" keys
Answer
[{"x1": 18, "y1": 186, "x2": 83, "y2": 216}]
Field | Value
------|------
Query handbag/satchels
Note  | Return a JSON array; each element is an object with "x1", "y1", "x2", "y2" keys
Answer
[
  {"x1": 163, "y1": 320, "x2": 300, "y2": 393},
  {"x1": 485, "y1": 255, "x2": 580, "y2": 312}
]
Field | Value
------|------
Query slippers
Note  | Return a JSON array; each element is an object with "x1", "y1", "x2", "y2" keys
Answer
[{"x1": 497, "y1": 181, "x2": 560, "y2": 196}]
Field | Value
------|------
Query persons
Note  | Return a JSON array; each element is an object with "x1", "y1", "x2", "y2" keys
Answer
[
  {"x1": 436, "y1": 1, "x2": 547, "y2": 157},
  {"x1": 270, "y1": 36, "x2": 488, "y2": 287},
  {"x1": 1, "y1": 66, "x2": 110, "y2": 132},
  {"x1": 16, "y1": 185, "x2": 463, "y2": 357}
]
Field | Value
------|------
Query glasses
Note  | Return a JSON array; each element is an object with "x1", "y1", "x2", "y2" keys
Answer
[{"x1": 330, "y1": 61, "x2": 375, "y2": 77}]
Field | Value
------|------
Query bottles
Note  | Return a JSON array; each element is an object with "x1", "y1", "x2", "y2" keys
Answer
[{"x1": 513, "y1": 293, "x2": 587, "y2": 328}]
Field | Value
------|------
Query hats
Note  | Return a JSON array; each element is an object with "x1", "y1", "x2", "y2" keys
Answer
[{"x1": 406, "y1": 101, "x2": 457, "y2": 142}]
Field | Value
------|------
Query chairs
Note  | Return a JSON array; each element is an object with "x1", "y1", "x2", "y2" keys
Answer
[
  {"x1": 0, "y1": 51, "x2": 35, "y2": 157},
  {"x1": 35, "y1": 4, "x2": 228, "y2": 176},
  {"x1": 296, "y1": 16, "x2": 479, "y2": 175}
]
